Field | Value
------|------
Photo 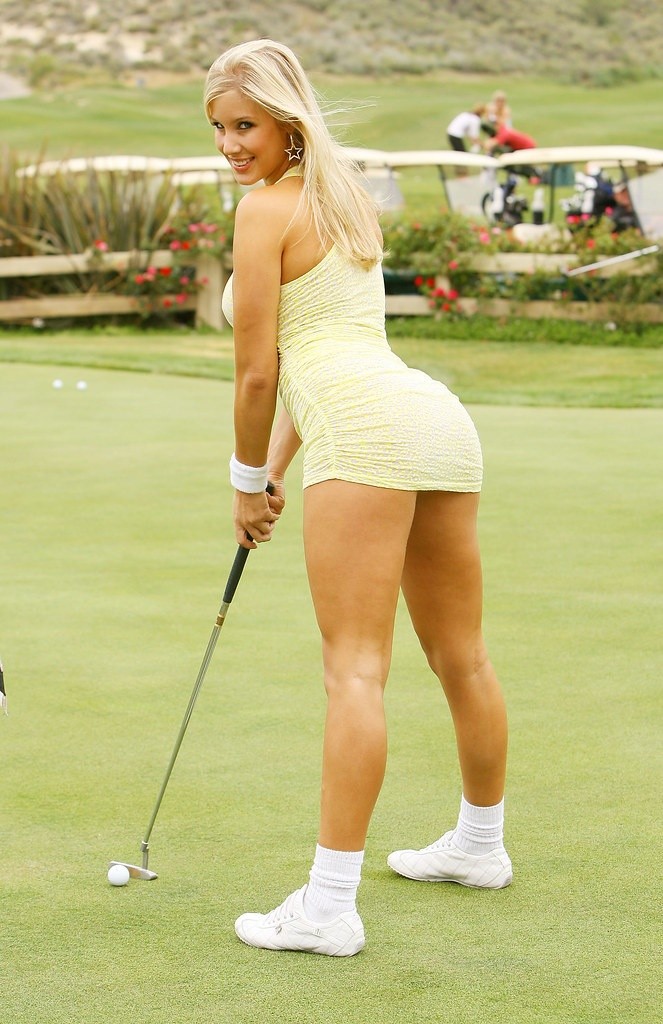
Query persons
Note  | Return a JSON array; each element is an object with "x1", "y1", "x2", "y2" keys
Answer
[
  {"x1": 202, "y1": 37, "x2": 515, "y2": 959},
  {"x1": 447, "y1": 90, "x2": 639, "y2": 235}
]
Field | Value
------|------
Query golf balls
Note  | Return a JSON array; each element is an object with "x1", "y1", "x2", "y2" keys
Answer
[{"x1": 103, "y1": 863, "x2": 131, "y2": 887}]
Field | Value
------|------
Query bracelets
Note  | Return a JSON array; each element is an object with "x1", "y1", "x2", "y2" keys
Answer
[{"x1": 227, "y1": 458, "x2": 270, "y2": 496}]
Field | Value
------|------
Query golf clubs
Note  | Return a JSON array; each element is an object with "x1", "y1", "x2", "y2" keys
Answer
[{"x1": 109, "y1": 484, "x2": 278, "y2": 882}]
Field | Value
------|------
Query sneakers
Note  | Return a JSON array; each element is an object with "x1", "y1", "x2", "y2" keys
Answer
[
  {"x1": 386, "y1": 826, "x2": 512, "y2": 890},
  {"x1": 234, "y1": 885, "x2": 365, "y2": 956}
]
